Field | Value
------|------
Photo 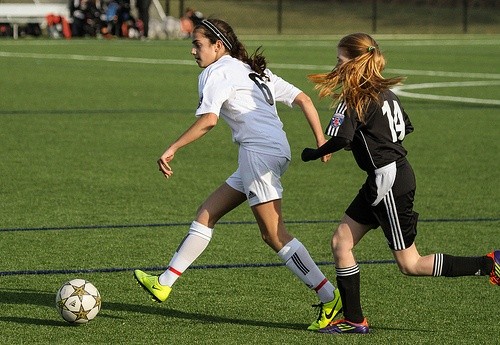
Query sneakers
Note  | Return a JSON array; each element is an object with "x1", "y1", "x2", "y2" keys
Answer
[
  {"x1": 133, "y1": 269, "x2": 172, "y2": 304},
  {"x1": 308, "y1": 288, "x2": 342, "y2": 331},
  {"x1": 319, "y1": 316, "x2": 369, "y2": 333},
  {"x1": 486, "y1": 250, "x2": 500, "y2": 287}
]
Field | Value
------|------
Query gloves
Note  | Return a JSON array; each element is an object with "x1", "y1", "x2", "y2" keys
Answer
[{"x1": 301, "y1": 136, "x2": 349, "y2": 162}]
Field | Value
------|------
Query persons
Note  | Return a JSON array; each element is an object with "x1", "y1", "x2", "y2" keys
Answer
[
  {"x1": 302, "y1": 32, "x2": 500, "y2": 335},
  {"x1": 134, "y1": 18, "x2": 344, "y2": 330},
  {"x1": 0, "y1": 0, "x2": 200, "y2": 41}
]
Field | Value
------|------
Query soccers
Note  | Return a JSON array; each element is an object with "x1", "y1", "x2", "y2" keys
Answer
[{"x1": 55, "y1": 278, "x2": 102, "y2": 325}]
